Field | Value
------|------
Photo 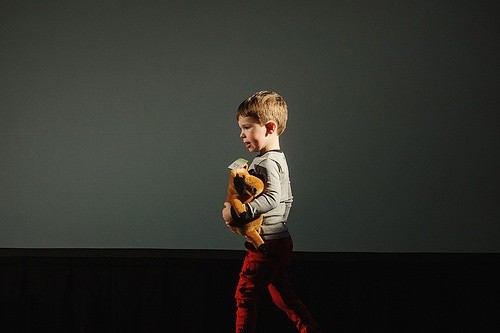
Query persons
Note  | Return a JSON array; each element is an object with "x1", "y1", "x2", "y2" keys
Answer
[{"x1": 221, "y1": 89, "x2": 323, "y2": 333}]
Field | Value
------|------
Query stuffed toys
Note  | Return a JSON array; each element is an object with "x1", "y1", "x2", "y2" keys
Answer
[{"x1": 226, "y1": 161, "x2": 270, "y2": 255}]
